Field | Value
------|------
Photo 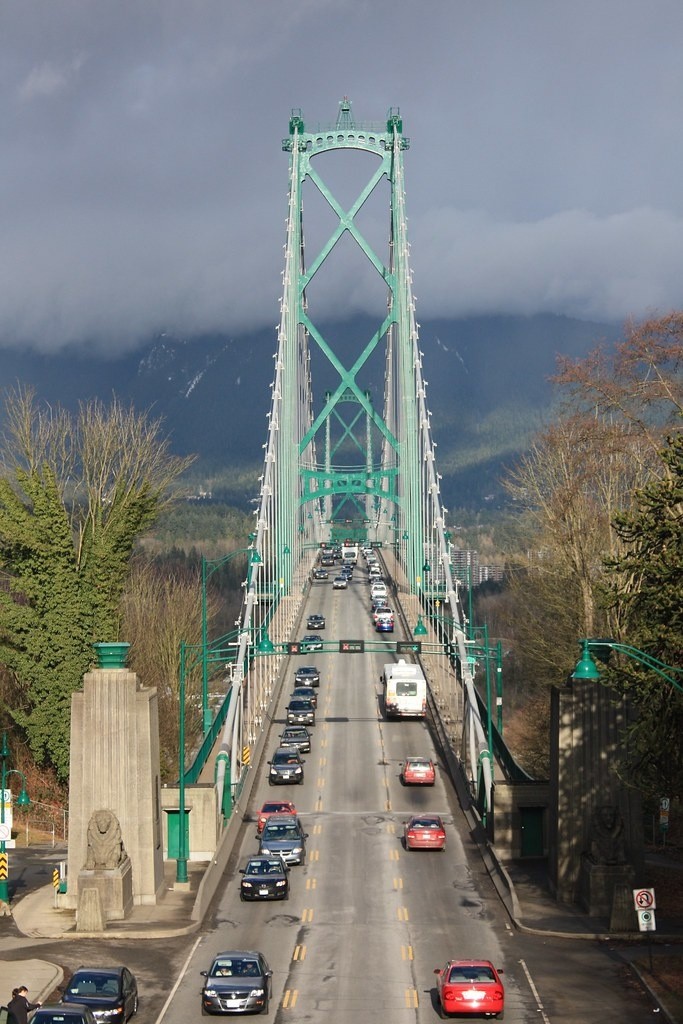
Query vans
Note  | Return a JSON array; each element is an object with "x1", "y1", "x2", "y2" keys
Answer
[{"x1": 267, "y1": 747, "x2": 305, "y2": 786}]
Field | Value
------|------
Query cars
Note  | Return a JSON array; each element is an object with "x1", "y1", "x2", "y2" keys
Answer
[
  {"x1": 313, "y1": 537, "x2": 397, "y2": 633},
  {"x1": 254, "y1": 821, "x2": 309, "y2": 866},
  {"x1": 278, "y1": 725, "x2": 313, "y2": 754},
  {"x1": 305, "y1": 614, "x2": 326, "y2": 631},
  {"x1": 289, "y1": 686, "x2": 319, "y2": 710},
  {"x1": 398, "y1": 756, "x2": 438, "y2": 787},
  {"x1": 199, "y1": 950, "x2": 273, "y2": 1016},
  {"x1": 433, "y1": 958, "x2": 506, "y2": 1021},
  {"x1": 293, "y1": 665, "x2": 321, "y2": 688},
  {"x1": 285, "y1": 699, "x2": 316, "y2": 727},
  {"x1": 257, "y1": 816, "x2": 304, "y2": 833},
  {"x1": 56, "y1": 965, "x2": 139, "y2": 1024},
  {"x1": 26, "y1": 1002, "x2": 98, "y2": 1024},
  {"x1": 239, "y1": 853, "x2": 291, "y2": 902},
  {"x1": 256, "y1": 799, "x2": 298, "y2": 835},
  {"x1": 402, "y1": 814, "x2": 447, "y2": 853}
]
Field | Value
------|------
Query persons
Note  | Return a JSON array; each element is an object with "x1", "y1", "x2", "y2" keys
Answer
[
  {"x1": 216, "y1": 967, "x2": 232, "y2": 976},
  {"x1": 6, "y1": 986, "x2": 42, "y2": 1024},
  {"x1": 243, "y1": 962, "x2": 257, "y2": 974}
]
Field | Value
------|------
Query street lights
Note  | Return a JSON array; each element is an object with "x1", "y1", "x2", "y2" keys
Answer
[
  {"x1": 199, "y1": 547, "x2": 264, "y2": 742},
  {"x1": 161, "y1": 626, "x2": 275, "y2": 892},
  {"x1": 414, "y1": 613, "x2": 495, "y2": 832},
  {"x1": 0, "y1": 729, "x2": 30, "y2": 916}
]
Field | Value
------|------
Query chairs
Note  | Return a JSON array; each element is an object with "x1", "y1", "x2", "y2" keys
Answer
[{"x1": 102, "y1": 980, "x2": 119, "y2": 995}]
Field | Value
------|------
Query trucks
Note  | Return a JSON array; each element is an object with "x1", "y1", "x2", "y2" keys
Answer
[{"x1": 379, "y1": 659, "x2": 427, "y2": 722}]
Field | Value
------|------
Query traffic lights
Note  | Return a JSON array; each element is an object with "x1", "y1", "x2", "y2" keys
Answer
[
  {"x1": 287, "y1": 641, "x2": 301, "y2": 655},
  {"x1": 344, "y1": 542, "x2": 355, "y2": 547},
  {"x1": 320, "y1": 542, "x2": 326, "y2": 549},
  {"x1": 396, "y1": 641, "x2": 421, "y2": 655},
  {"x1": 339, "y1": 639, "x2": 365, "y2": 654},
  {"x1": 371, "y1": 542, "x2": 382, "y2": 548}
]
